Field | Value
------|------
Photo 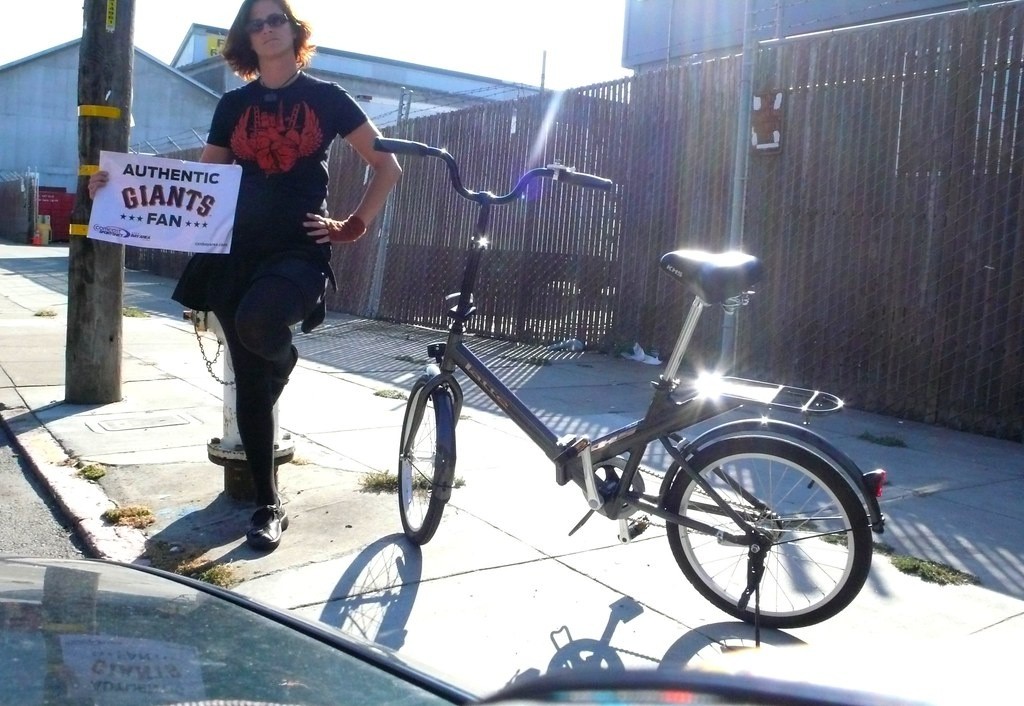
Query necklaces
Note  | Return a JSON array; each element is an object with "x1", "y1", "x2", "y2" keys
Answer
[{"x1": 258, "y1": 70, "x2": 298, "y2": 89}]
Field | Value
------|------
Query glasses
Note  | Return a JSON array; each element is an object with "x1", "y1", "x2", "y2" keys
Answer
[{"x1": 244, "y1": 12, "x2": 289, "y2": 36}]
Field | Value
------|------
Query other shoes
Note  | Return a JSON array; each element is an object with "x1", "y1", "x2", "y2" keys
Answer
[
  {"x1": 270, "y1": 345, "x2": 298, "y2": 406},
  {"x1": 246, "y1": 496, "x2": 289, "y2": 551}
]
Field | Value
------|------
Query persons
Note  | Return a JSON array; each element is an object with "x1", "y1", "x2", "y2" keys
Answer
[{"x1": 90, "y1": 0, "x2": 400, "y2": 551}]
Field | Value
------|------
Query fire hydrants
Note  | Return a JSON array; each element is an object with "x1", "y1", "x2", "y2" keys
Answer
[{"x1": 183, "y1": 305, "x2": 295, "y2": 502}]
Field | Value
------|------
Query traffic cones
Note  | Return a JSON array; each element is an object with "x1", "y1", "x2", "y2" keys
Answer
[{"x1": 31, "y1": 230, "x2": 43, "y2": 246}]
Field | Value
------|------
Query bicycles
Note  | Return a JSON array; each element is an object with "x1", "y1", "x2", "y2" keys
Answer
[{"x1": 372, "y1": 135, "x2": 887, "y2": 650}]
